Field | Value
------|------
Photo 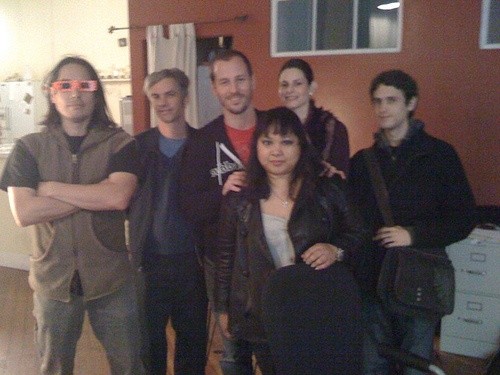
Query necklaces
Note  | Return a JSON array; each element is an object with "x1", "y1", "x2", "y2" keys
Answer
[{"x1": 273, "y1": 191, "x2": 289, "y2": 208}]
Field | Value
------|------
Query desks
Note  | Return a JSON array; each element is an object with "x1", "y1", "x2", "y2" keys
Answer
[{"x1": 0, "y1": 145, "x2": 31, "y2": 272}]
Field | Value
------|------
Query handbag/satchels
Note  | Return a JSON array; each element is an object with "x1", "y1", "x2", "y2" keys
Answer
[
  {"x1": 262, "y1": 262, "x2": 365, "y2": 375},
  {"x1": 378, "y1": 248, "x2": 457, "y2": 317}
]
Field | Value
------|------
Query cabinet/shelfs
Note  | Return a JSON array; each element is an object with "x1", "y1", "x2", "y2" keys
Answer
[{"x1": 439, "y1": 225, "x2": 500, "y2": 359}]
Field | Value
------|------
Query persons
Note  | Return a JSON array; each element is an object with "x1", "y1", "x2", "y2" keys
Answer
[
  {"x1": 345, "y1": 69, "x2": 479, "y2": 375},
  {"x1": 128, "y1": 67, "x2": 208, "y2": 375},
  {"x1": 276, "y1": 58, "x2": 350, "y2": 172},
  {"x1": 0, "y1": 54, "x2": 149, "y2": 375},
  {"x1": 179, "y1": 48, "x2": 347, "y2": 375},
  {"x1": 212, "y1": 106, "x2": 374, "y2": 375}
]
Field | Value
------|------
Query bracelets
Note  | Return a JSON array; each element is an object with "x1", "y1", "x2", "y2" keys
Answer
[{"x1": 337, "y1": 248, "x2": 344, "y2": 261}]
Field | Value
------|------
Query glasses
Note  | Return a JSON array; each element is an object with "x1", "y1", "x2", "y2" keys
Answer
[{"x1": 49, "y1": 80, "x2": 100, "y2": 93}]
